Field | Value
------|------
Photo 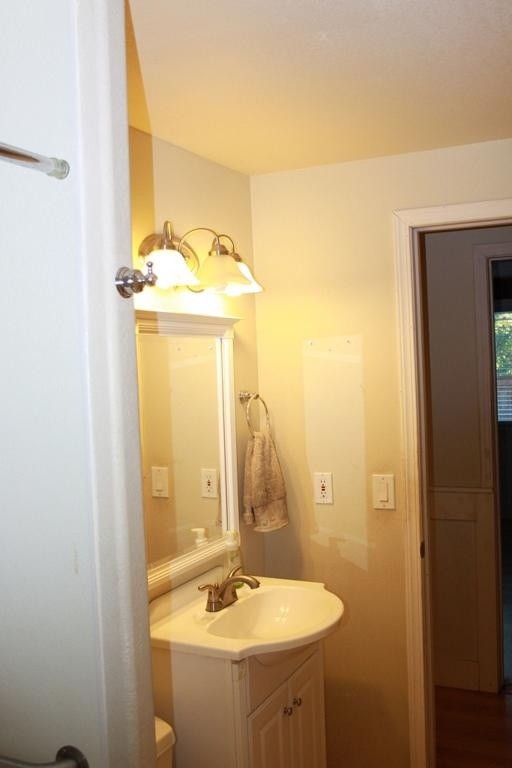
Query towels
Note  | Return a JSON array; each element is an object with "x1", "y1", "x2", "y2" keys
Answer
[{"x1": 243, "y1": 431, "x2": 290, "y2": 534}]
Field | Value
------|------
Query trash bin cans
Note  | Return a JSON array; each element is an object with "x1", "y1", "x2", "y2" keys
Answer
[{"x1": 155, "y1": 717, "x2": 176, "y2": 768}]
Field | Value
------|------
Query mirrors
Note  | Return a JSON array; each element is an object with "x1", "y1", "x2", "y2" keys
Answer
[{"x1": 133, "y1": 308, "x2": 242, "y2": 604}]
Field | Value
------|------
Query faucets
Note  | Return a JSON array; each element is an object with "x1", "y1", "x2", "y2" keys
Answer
[{"x1": 218, "y1": 573, "x2": 260, "y2": 608}]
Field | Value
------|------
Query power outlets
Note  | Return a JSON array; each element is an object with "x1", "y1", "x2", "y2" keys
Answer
[
  {"x1": 313, "y1": 472, "x2": 333, "y2": 503},
  {"x1": 200, "y1": 468, "x2": 218, "y2": 499}
]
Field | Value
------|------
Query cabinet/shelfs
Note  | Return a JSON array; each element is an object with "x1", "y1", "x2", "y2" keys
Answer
[{"x1": 170, "y1": 644, "x2": 326, "y2": 768}]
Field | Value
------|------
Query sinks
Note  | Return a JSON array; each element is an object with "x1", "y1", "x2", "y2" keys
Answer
[{"x1": 150, "y1": 576, "x2": 344, "y2": 662}]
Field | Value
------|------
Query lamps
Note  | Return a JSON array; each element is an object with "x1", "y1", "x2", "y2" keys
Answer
[{"x1": 136, "y1": 220, "x2": 262, "y2": 301}]
